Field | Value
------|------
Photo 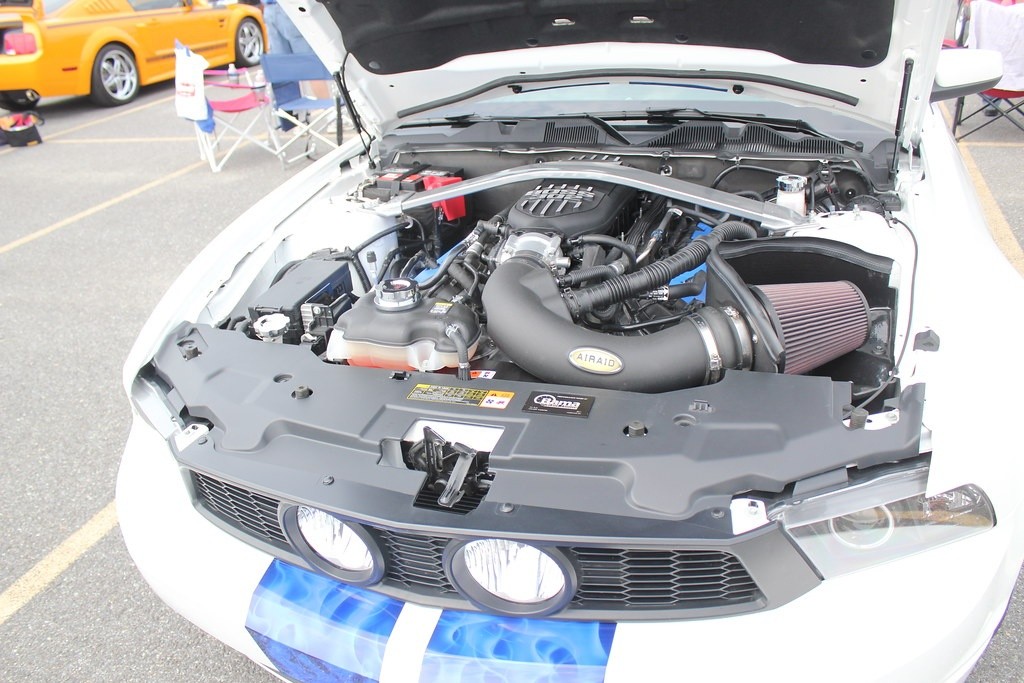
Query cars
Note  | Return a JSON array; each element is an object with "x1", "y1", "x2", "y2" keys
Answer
[
  {"x1": 0, "y1": 0, "x2": 268, "y2": 112},
  {"x1": 111, "y1": 0, "x2": 1024, "y2": 683}
]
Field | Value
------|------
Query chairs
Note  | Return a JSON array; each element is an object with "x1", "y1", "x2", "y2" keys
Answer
[
  {"x1": 174, "y1": 47, "x2": 288, "y2": 175},
  {"x1": 262, "y1": 50, "x2": 349, "y2": 162}
]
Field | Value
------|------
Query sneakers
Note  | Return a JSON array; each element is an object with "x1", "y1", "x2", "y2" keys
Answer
[{"x1": 326, "y1": 115, "x2": 355, "y2": 135}]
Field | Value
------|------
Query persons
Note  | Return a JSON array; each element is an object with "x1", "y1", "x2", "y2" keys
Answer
[{"x1": 261, "y1": 0, "x2": 356, "y2": 134}]
